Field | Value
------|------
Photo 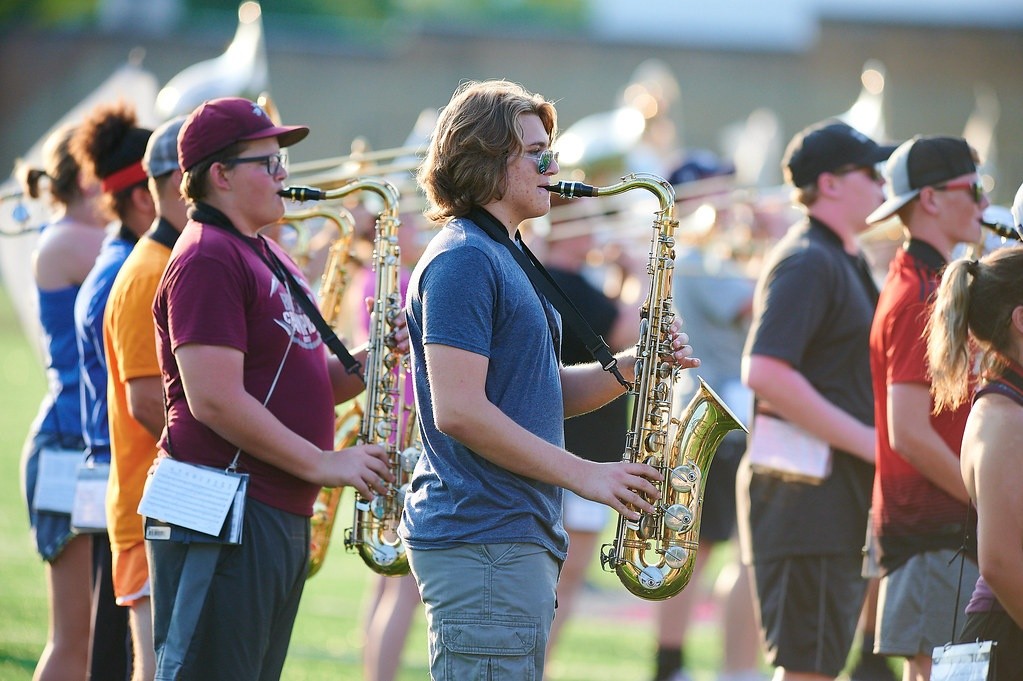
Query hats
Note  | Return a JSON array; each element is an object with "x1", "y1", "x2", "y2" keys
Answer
[
  {"x1": 141, "y1": 113, "x2": 191, "y2": 177},
  {"x1": 176, "y1": 97, "x2": 310, "y2": 173},
  {"x1": 783, "y1": 119, "x2": 899, "y2": 186},
  {"x1": 865, "y1": 136, "x2": 981, "y2": 223},
  {"x1": 668, "y1": 157, "x2": 739, "y2": 199}
]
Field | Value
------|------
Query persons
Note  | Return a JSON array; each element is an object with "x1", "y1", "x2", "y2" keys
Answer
[
  {"x1": 141, "y1": 98, "x2": 409, "y2": 681},
  {"x1": 393, "y1": 81, "x2": 701, "y2": 680},
  {"x1": 363, "y1": 106, "x2": 1023, "y2": 681},
  {"x1": 24, "y1": 106, "x2": 190, "y2": 681}
]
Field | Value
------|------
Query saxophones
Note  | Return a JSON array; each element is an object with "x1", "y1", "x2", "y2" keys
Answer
[
  {"x1": 278, "y1": 175, "x2": 419, "y2": 576},
  {"x1": 545, "y1": 172, "x2": 751, "y2": 600},
  {"x1": 275, "y1": 205, "x2": 363, "y2": 576}
]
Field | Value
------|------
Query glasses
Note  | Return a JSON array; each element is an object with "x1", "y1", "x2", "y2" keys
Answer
[
  {"x1": 829, "y1": 163, "x2": 881, "y2": 181},
  {"x1": 507, "y1": 149, "x2": 559, "y2": 174},
  {"x1": 933, "y1": 181, "x2": 985, "y2": 203},
  {"x1": 209, "y1": 154, "x2": 288, "y2": 175}
]
